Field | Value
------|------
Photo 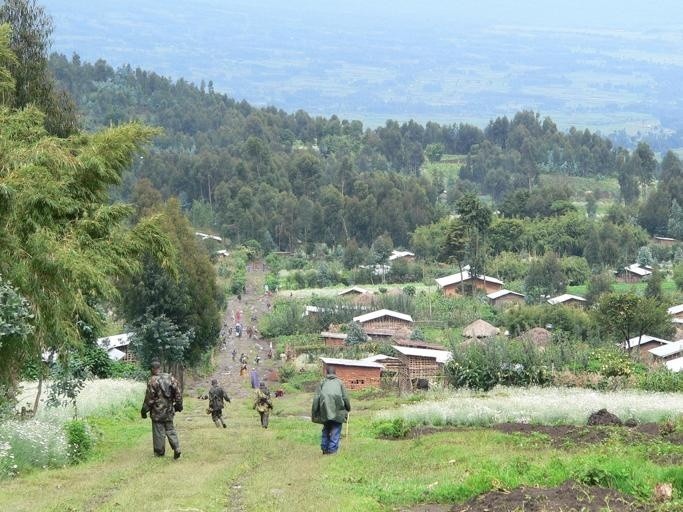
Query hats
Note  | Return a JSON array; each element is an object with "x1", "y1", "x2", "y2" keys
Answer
[{"x1": 147, "y1": 360, "x2": 160, "y2": 370}]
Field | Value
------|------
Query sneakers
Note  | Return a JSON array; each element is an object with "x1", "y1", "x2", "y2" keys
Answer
[{"x1": 174, "y1": 450, "x2": 181, "y2": 459}]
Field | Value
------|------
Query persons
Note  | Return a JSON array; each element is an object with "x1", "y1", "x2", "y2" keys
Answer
[
  {"x1": 208, "y1": 380, "x2": 231, "y2": 428},
  {"x1": 221, "y1": 283, "x2": 287, "y2": 397},
  {"x1": 313, "y1": 366, "x2": 350, "y2": 454},
  {"x1": 252, "y1": 381, "x2": 272, "y2": 428},
  {"x1": 141, "y1": 361, "x2": 183, "y2": 459}
]
